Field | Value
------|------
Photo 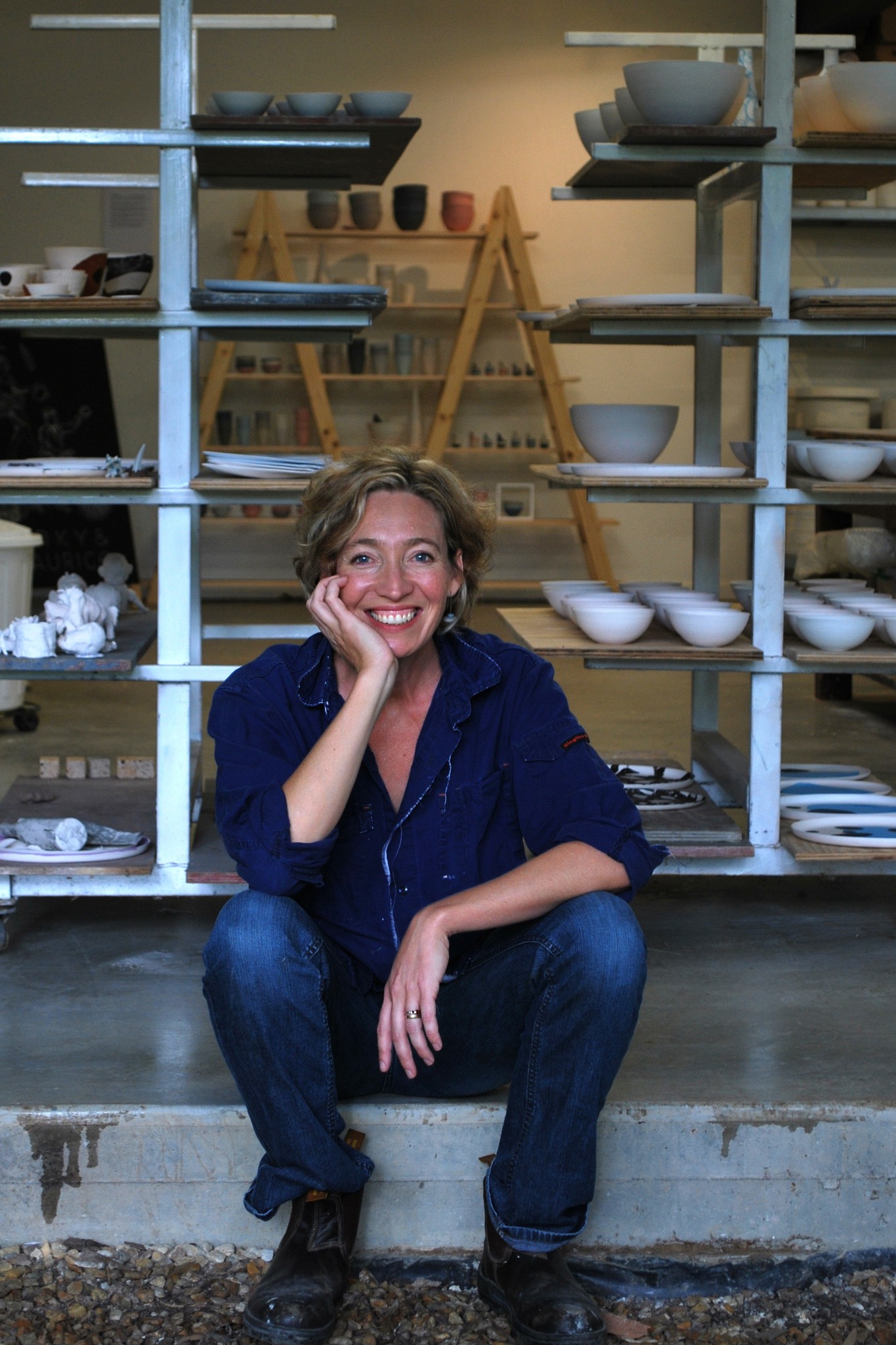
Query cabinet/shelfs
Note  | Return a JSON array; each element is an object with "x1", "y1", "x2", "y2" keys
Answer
[{"x1": 0, "y1": 0, "x2": 896, "y2": 895}]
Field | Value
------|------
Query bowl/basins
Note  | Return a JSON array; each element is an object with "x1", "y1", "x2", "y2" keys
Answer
[
  {"x1": 538, "y1": 579, "x2": 750, "y2": 646},
  {"x1": 568, "y1": 403, "x2": 679, "y2": 463},
  {"x1": 728, "y1": 438, "x2": 896, "y2": 482},
  {"x1": 572, "y1": 58, "x2": 747, "y2": 156},
  {"x1": 729, "y1": 577, "x2": 896, "y2": 651},
  {"x1": 306, "y1": 183, "x2": 476, "y2": 231},
  {"x1": 366, "y1": 420, "x2": 405, "y2": 444},
  {"x1": 503, "y1": 499, "x2": 524, "y2": 515},
  {"x1": 204, "y1": 91, "x2": 413, "y2": 117},
  {"x1": 793, "y1": 61, "x2": 896, "y2": 138}
]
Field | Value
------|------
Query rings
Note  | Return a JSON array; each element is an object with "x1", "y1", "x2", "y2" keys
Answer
[{"x1": 404, "y1": 1010, "x2": 421, "y2": 1019}]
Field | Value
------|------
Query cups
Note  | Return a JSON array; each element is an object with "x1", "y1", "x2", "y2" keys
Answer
[
  {"x1": 198, "y1": 503, "x2": 296, "y2": 518},
  {"x1": 216, "y1": 405, "x2": 314, "y2": 446},
  {"x1": 235, "y1": 332, "x2": 440, "y2": 375},
  {"x1": 0, "y1": 243, "x2": 154, "y2": 300}
]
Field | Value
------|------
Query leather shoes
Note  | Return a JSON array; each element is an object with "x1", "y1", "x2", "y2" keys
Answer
[
  {"x1": 477, "y1": 1195, "x2": 607, "y2": 1345},
  {"x1": 243, "y1": 1130, "x2": 366, "y2": 1345}
]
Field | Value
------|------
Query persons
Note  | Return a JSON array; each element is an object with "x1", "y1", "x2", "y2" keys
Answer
[{"x1": 201, "y1": 452, "x2": 662, "y2": 1345}]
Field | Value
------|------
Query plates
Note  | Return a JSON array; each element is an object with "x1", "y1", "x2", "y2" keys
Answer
[
  {"x1": 605, "y1": 763, "x2": 706, "y2": 811},
  {"x1": 1, "y1": 455, "x2": 158, "y2": 476},
  {"x1": 0, "y1": 837, "x2": 151, "y2": 864},
  {"x1": 515, "y1": 292, "x2": 753, "y2": 321},
  {"x1": 557, "y1": 462, "x2": 747, "y2": 478},
  {"x1": 205, "y1": 277, "x2": 385, "y2": 293},
  {"x1": 201, "y1": 448, "x2": 326, "y2": 480},
  {"x1": 780, "y1": 762, "x2": 896, "y2": 847},
  {"x1": 789, "y1": 286, "x2": 896, "y2": 298},
  {"x1": 792, "y1": 206, "x2": 896, "y2": 220}
]
